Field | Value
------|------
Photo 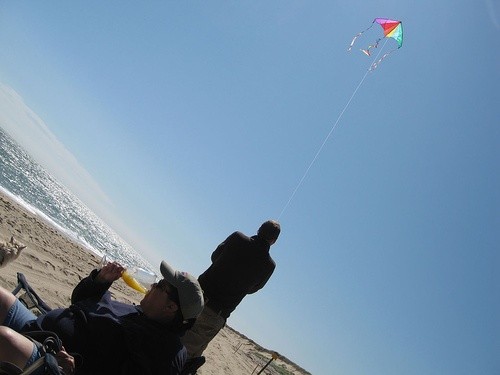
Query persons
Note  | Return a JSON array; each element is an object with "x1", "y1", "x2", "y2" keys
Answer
[
  {"x1": 182, "y1": 221, "x2": 282, "y2": 368},
  {"x1": 0, "y1": 262, "x2": 205, "y2": 375}
]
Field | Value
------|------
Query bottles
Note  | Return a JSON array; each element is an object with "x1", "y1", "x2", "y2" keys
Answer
[{"x1": 160, "y1": 260, "x2": 204, "y2": 325}]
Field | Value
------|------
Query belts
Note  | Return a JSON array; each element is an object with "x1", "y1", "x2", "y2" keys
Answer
[{"x1": 100, "y1": 255, "x2": 156, "y2": 294}]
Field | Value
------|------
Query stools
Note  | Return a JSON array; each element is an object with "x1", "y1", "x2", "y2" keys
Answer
[{"x1": 204, "y1": 297, "x2": 230, "y2": 318}]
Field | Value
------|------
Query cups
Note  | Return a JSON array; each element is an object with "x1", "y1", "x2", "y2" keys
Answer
[{"x1": 157, "y1": 279, "x2": 176, "y2": 300}]
